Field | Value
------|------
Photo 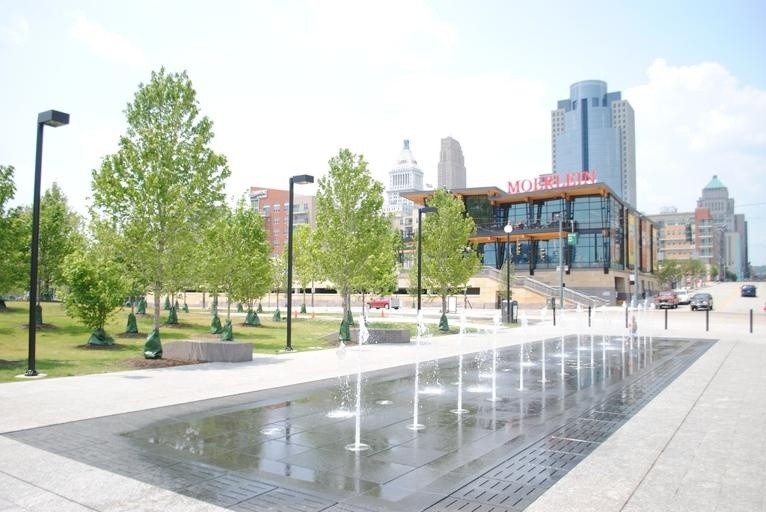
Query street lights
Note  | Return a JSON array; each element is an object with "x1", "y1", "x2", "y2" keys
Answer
[
  {"x1": 24, "y1": 110, "x2": 70, "y2": 376},
  {"x1": 285, "y1": 174, "x2": 314, "y2": 350},
  {"x1": 417, "y1": 206, "x2": 438, "y2": 336},
  {"x1": 503, "y1": 224, "x2": 513, "y2": 322}
]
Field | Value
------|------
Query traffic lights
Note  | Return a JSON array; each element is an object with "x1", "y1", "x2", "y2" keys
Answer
[
  {"x1": 685, "y1": 223, "x2": 693, "y2": 242},
  {"x1": 540, "y1": 248, "x2": 545, "y2": 260},
  {"x1": 516, "y1": 241, "x2": 523, "y2": 256}
]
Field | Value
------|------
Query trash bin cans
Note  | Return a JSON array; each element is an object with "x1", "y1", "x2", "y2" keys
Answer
[{"x1": 501, "y1": 300, "x2": 518, "y2": 323}]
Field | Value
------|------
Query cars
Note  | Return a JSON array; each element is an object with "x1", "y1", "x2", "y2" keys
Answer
[
  {"x1": 689, "y1": 293, "x2": 712, "y2": 310},
  {"x1": 656, "y1": 287, "x2": 689, "y2": 308},
  {"x1": 742, "y1": 283, "x2": 755, "y2": 297},
  {"x1": 367, "y1": 295, "x2": 397, "y2": 309}
]
